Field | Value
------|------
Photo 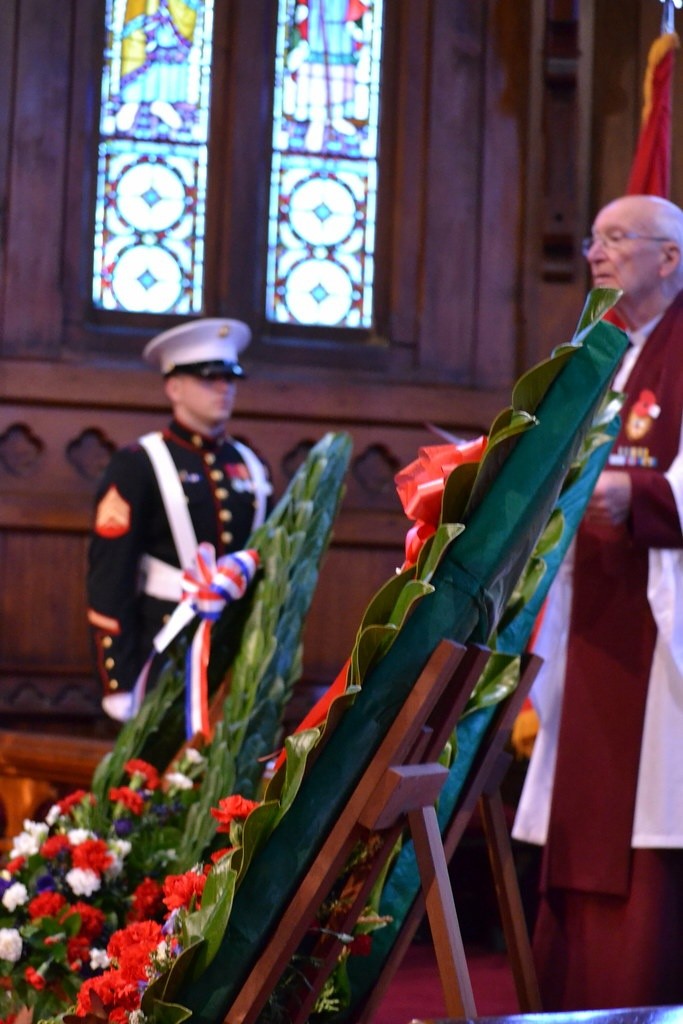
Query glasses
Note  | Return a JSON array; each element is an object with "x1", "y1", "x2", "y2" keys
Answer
[{"x1": 580, "y1": 227, "x2": 678, "y2": 257}]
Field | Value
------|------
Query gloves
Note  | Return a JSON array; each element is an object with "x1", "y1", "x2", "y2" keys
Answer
[{"x1": 101, "y1": 692, "x2": 133, "y2": 722}]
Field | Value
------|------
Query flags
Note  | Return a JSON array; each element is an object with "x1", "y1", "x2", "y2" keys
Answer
[{"x1": 616, "y1": 28, "x2": 678, "y2": 206}]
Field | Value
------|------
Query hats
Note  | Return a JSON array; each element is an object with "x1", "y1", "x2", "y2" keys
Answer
[{"x1": 141, "y1": 317, "x2": 253, "y2": 380}]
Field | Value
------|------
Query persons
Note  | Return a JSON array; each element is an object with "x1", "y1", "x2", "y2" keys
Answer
[
  {"x1": 87, "y1": 318, "x2": 278, "y2": 777},
  {"x1": 507, "y1": 192, "x2": 682, "y2": 1017}
]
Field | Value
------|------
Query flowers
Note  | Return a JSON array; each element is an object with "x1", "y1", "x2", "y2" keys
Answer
[
  {"x1": 311, "y1": 919, "x2": 372, "y2": 964},
  {"x1": 0, "y1": 758, "x2": 260, "y2": 1024}
]
